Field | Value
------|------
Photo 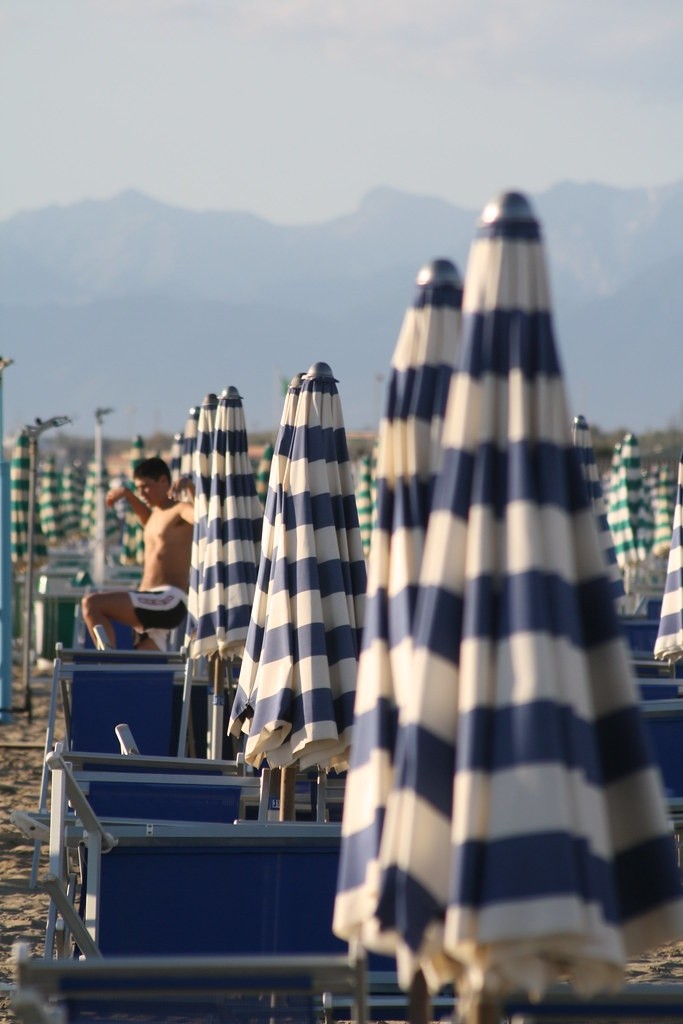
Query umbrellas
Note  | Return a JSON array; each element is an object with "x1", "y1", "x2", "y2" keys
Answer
[
  {"x1": 39, "y1": 450, "x2": 118, "y2": 541},
  {"x1": 331, "y1": 190, "x2": 683, "y2": 1024},
  {"x1": 169, "y1": 406, "x2": 201, "y2": 480},
  {"x1": 116, "y1": 434, "x2": 147, "y2": 565},
  {"x1": 180, "y1": 387, "x2": 263, "y2": 759},
  {"x1": 226, "y1": 362, "x2": 366, "y2": 820},
  {"x1": 10, "y1": 431, "x2": 49, "y2": 563}
]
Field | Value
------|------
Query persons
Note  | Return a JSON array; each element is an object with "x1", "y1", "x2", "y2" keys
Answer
[{"x1": 81, "y1": 457, "x2": 195, "y2": 651}]
